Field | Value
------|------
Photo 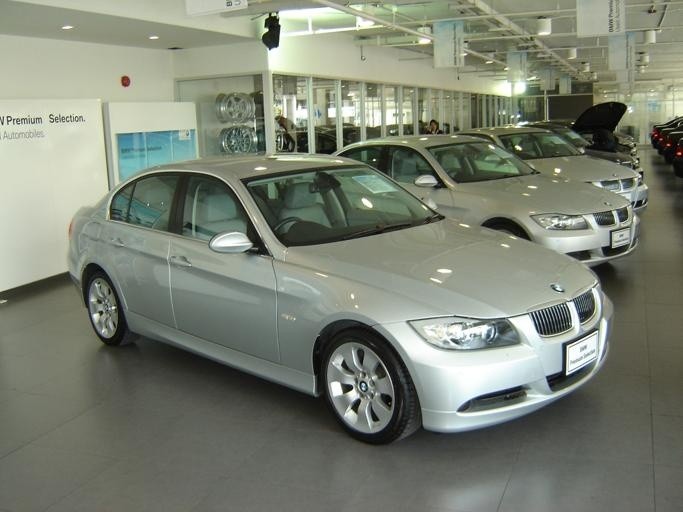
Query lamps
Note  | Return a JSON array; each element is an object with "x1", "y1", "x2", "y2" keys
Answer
[
  {"x1": 636, "y1": 65, "x2": 645, "y2": 76},
  {"x1": 592, "y1": 71, "x2": 598, "y2": 79},
  {"x1": 504, "y1": 60, "x2": 510, "y2": 70},
  {"x1": 536, "y1": 19, "x2": 553, "y2": 36},
  {"x1": 567, "y1": 48, "x2": 578, "y2": 60},
  {"x1": 641, "y1": 29, "x2": 657, "y2": 47},
  {"x1": 530, "y1": 70, "x2": 535, "y2": 78},
  {"x1": 415, "y1": 24, "x2": 431, "y2": 47},
  {"x1": 581, "y1": 63, "x2": 589, "y2": 73},
  {"x1": 484, "y1": 52, "x2": 496, "y2": 63},
  {"x1": 462, "y1": 43, "x2": 468, "y2": 56},
  {"x1": 640, "y1": 55, "x2": 650, "y2": 64}
]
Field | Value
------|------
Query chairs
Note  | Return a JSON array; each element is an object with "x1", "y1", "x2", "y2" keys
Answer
[
  {"x1": 138, "y1": 187, "x2": 190, "y2": 234},
  {"x1": 193, "y1": 194, "x2": 247, "y2": 248},
  {"x1": 279, "y1": 181, "x2": 331, "y2": 232},
  {"x1": 517, "y1": 139, "x2": 535, "y2": 157},
  {"x1": 396, "y1": 158, "x2": 418, "y2": 183}
]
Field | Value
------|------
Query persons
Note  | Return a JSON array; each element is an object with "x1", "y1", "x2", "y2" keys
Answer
[
  {"x1": 426, "y1": 120, "x2": 443, "y2": 134},
  {"x1": 591, "y1": 125, "x2": 616, "y2": 152}
]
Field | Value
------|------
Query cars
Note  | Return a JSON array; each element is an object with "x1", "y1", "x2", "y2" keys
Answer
[
  {"x1": 511, "y1": 101, "x2": 643, "y2": 182},
  {"x1": 66, "y1": 152, "x2": 613, "y2": 449},
  {"x1": 329, "y1": 134, "x2": 639, "y2": 269},
  {"x1": 274, "y1": 116, "x2": 461, "y2": 155},
  {"x1": 649, "y1": 116, "x2": 682, "y2": 180},
  {"x1": 452, "y1": 125, "x2": 648, "y2": 217}
]
